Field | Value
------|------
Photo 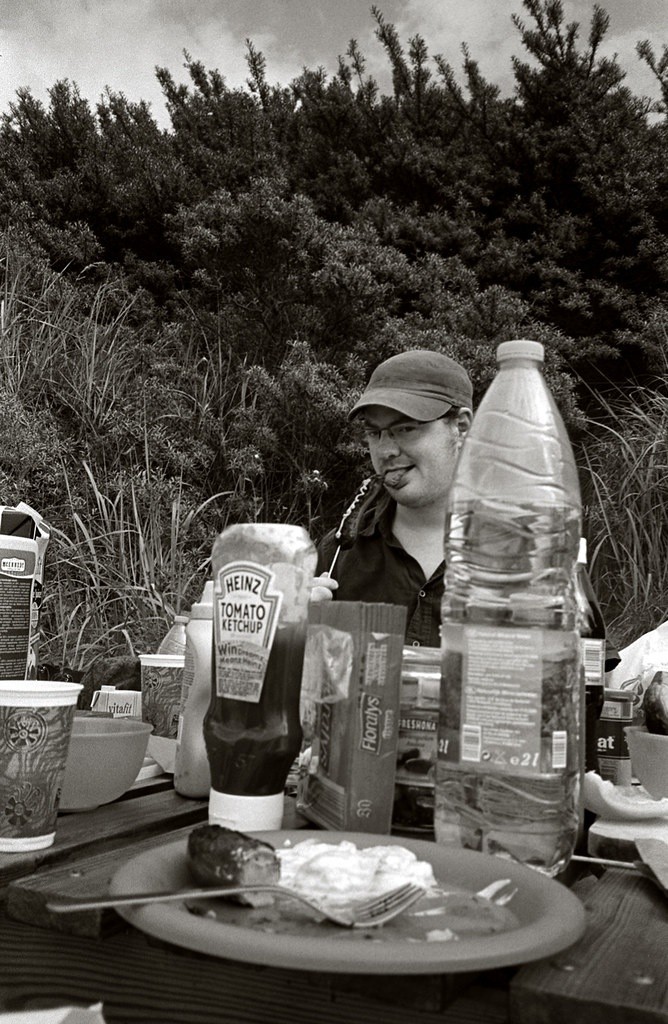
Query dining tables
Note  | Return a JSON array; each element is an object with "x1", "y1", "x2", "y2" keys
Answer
[{"x1": 0, "y1": 738, "x2": 668, "y2": 1024}]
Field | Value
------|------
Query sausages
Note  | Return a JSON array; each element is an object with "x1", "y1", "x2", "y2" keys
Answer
[{"x1": 187, "y1": 824, "x2": 281, "y2": 908}]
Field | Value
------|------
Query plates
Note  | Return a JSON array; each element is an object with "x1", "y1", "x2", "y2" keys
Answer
[{"x1": 109, "y1": 831, "x2": 587, "y2": 974}]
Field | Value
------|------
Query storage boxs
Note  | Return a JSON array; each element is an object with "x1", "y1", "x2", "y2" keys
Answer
[
  {"x1": 305, "y1": 598, "x2": 408, "y2": 844},
  {"x1": 0, "y1": 502, "x2": 53, "y2": 681}
]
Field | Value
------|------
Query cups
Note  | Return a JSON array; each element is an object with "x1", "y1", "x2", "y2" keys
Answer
[
  {"x1": 0, "y1": 680, "x2": 84, "y2": 851},
  {"x1": 138, "y1": 654, "x2": 186, "y2": 739}
]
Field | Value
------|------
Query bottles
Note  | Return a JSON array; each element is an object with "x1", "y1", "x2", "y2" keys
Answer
[
  {"x1": 596, "y1": 688, "x2": 634, "y2": 785},
  {"x1": 575, "y1": 537, "x2": 606, "y2": 775},
  {"x1": 202, "y1": 523, "x2": 318, "y2": 831},
  {"x1": 434, "y1": 340, "x2": 585, "y2": 878},
  {"x1": 173, "y1": 581, "x2": 214, "y2": 797},
  {"x1": 156, "y1": 616, "x2": 190, "y2": 655}
]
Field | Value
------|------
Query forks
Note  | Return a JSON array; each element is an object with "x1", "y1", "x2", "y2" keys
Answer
[{"x1": 46, "y1": 883, "x2": 426, "y2": 927}]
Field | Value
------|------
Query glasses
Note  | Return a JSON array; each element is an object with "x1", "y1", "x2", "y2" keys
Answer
[{"x1": 358, "y1": 410, "x2": 460, "y2": 447}]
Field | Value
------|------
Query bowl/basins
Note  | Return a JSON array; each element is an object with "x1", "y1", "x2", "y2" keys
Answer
[
  {"x1": 624, "y1": 726, "x2": 668, "y2": 801},
  {"x1": 57, "y1": 717, "x2": 153, "y2": 812}
]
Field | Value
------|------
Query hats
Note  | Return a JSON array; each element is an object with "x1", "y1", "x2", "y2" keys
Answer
[{"x1": 347, "y1": 350, "x2": 473, "y2": 424}]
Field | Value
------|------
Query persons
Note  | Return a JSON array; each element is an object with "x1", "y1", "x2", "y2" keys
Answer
[{"x1": 310, "y1": 350, "x2": 622, "y2": 674}]
